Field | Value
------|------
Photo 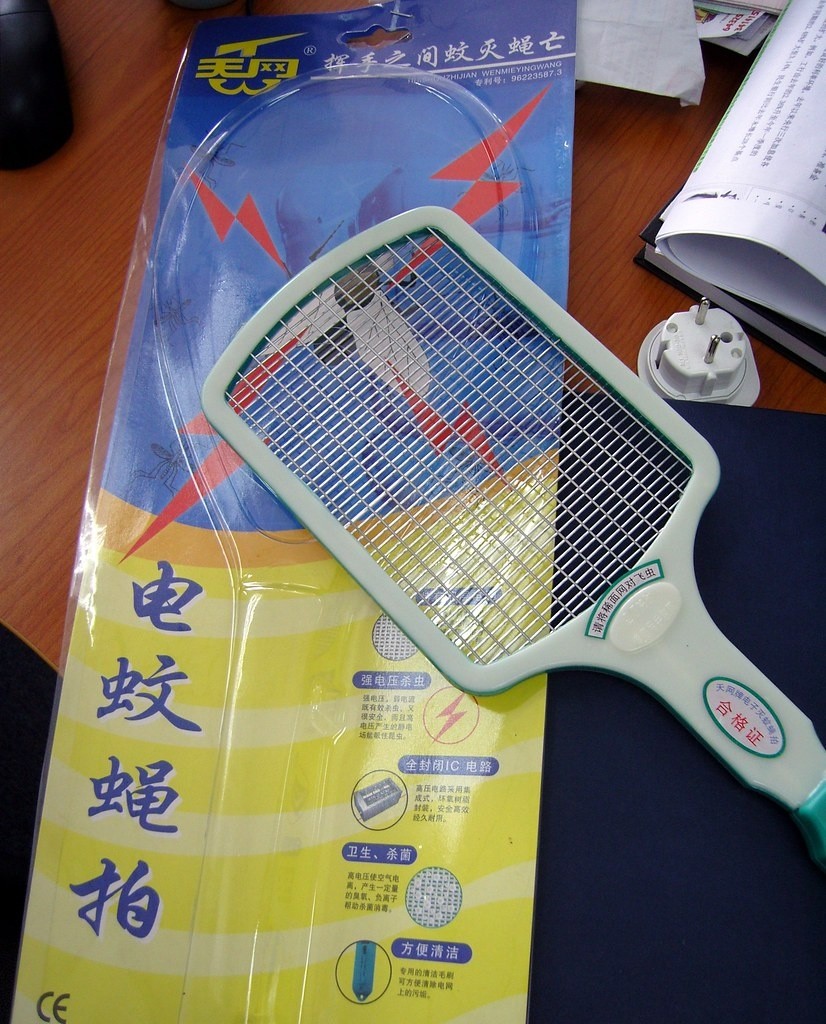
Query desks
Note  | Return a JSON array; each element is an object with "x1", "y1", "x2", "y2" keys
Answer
[{"x1": 0, "y1": 0, "x2": 826, "y2": 1024}]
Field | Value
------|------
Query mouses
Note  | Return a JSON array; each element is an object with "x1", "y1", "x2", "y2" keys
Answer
[{"x1": 0, "y1": 0, "x2": 74, "y2": 172}]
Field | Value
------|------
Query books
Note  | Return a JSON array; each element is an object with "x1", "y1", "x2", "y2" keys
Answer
[{"x1": 632, "y1": 172, "x2": 826, "y2": 385}]
